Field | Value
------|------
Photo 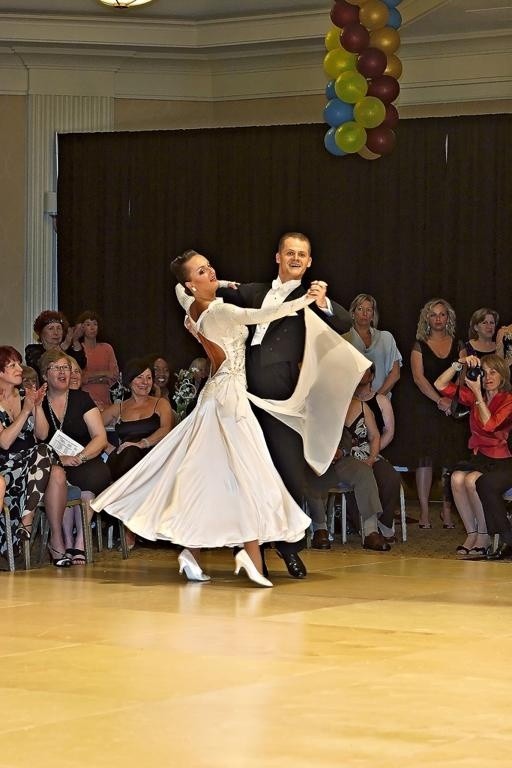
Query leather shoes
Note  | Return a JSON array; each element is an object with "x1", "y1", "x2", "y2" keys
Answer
[
  {"x1": 310, "y1": 528, "x2": 333, "y2": 550},
  {"x1": 487, "y1": 542, "x2": 512, "y2": 561},
  {"x1": 277, "y1": 544, "x2": 311, "y2": 579},
  {"x1": 362, "y1": 531, "x2": 391, "y2": 551}
]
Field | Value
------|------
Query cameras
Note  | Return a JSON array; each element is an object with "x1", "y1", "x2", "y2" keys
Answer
[{"x1": 466, "y1": 365, "x2": 484, "y2": 379}]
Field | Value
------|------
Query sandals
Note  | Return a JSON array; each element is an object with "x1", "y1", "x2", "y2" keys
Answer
[
  {"x1": 65, "y1": 548, "x2": 74, "y2": 560},
  {"x1": 73, "y1": 547, "x2": 88, "y2": 566}
]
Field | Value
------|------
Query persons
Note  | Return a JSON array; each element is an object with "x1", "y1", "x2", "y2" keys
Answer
[
  {"x1": 89, "y1": 249, "x2": 372, "y2": 578},
  {"x1": 344, "y1": 398, "x2": 400, "y2": 544},
  {"x1": 303, "y1": 427, "x2": 390, "y2": 551},
  {"x1": 1, "y1": 310, "x2": 210, "y2": 574},
  {"x1": 340, "y1": 294, "x2": 403, "y2": 401},
  {"x1": 410, "y1": 298, "x2": 512, "y2": 555},
  {"x1": 185, "y1": 232, "x2": 351, "y2": 586},
  {"x1": 350, "y1": 363, "x2": 395, "y2": 461}
]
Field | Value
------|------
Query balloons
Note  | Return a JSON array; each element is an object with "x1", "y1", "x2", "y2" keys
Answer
[{"x1": 323, "y1": 0, "x2": 404, "y2": 161}]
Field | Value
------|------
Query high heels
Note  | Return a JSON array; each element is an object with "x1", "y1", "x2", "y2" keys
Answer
[
  {"x1": 468, "y1": 531, "x2": 492, "y2": 556},
  {"x1": 174, "y1": 549, "x2": 213, "y2": 583},
  {"x1": 116, "y1": 531, "x2": 138, "y2": 552},
  {"x1": 14, "y1": 515, "x2": 35, "y2": 540},
  {"x1": 231, "y1": 549, "x2": 275, "y2": 588},
  {"x1": 48, "y1": 543, "x2": 72, "y2": 567},
  {"x1": 456, "y1": 531, "x2": 477, "y2": 556},
  {"x1": 438, "y1": 510, "x2": 456, "y2": 530},
  {"x1": 418, "y1": 514, "x2": 433, "y2": 530}
]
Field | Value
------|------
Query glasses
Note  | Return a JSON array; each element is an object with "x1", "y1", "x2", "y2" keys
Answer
[{"x1": 47, "y1": 363, "x2": 73, "y2": 372}]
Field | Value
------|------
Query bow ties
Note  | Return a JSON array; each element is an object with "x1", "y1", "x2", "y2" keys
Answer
[{"x1": 269, "y1": 278, "x2": 293, "y2": 295}]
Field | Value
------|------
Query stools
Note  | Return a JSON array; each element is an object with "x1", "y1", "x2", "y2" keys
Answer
[{"x1": 493, "y1": 488, "x2": 512, "y2": 553}]
